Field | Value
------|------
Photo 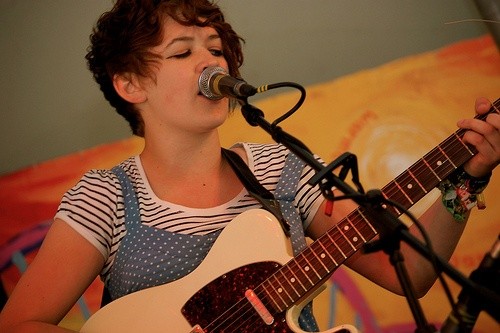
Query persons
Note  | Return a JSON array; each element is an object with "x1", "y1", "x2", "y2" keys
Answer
[{"x1": 0, "y1": 0, "x2": 500, "y2": 333}]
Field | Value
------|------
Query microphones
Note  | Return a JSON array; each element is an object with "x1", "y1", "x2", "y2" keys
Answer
[
  {"x1": 440, "y1": 233, "x2": 500, "y2": 333},
  {"x1": 198, "y1": 65, "x2": 256, "y2": 99}
]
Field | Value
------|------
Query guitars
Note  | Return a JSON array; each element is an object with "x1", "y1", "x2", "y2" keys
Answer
[{"x1": 79, "y1": 99, "x2": 500, "y2": 333}]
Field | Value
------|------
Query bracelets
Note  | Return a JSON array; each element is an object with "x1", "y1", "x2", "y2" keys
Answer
[{"x1": 437, "y1": 169, "x2": 493, "y2": 223}]
